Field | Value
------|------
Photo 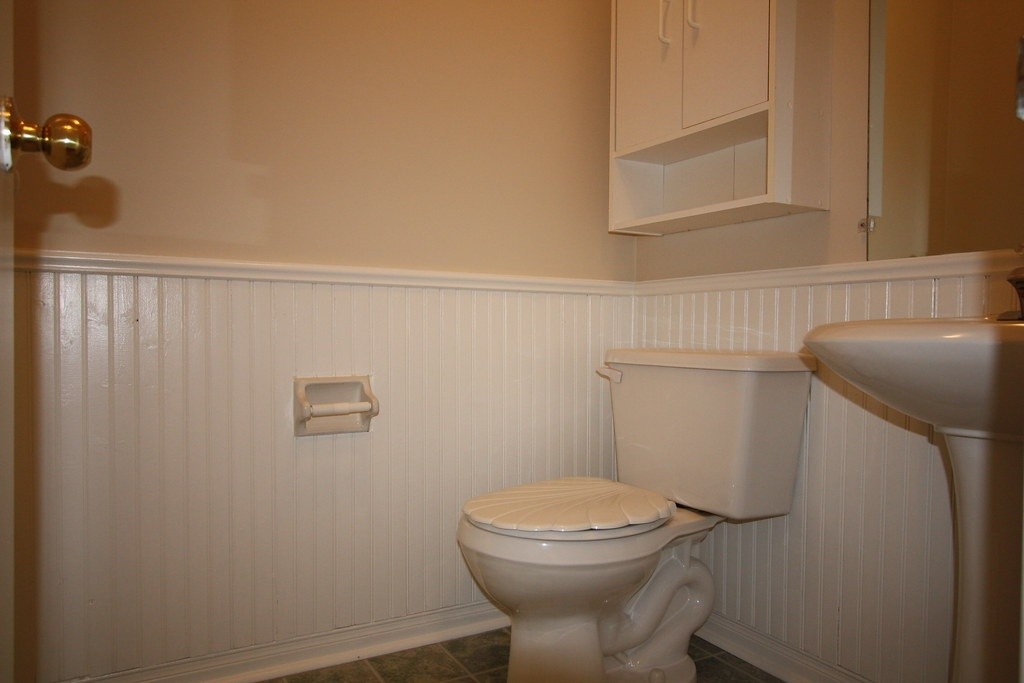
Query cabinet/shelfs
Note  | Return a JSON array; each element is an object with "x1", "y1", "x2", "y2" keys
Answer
[{"x1": 608, "y1": 0, "x2": 832, "y2": 237}]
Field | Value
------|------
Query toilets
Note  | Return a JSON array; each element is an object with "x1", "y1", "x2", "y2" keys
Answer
[{"x1": 456, "y1": 347, "x2": 819, "y2": 683}]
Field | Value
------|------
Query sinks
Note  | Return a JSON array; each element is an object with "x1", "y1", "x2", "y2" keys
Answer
[{"x1": 803, "y1": 315, "x2": 1024, "y2": 443}]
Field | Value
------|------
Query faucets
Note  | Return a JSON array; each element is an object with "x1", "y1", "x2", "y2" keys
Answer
[{"x1": 996, "y1": 266, "x2": 1024, "y2": 321}]
